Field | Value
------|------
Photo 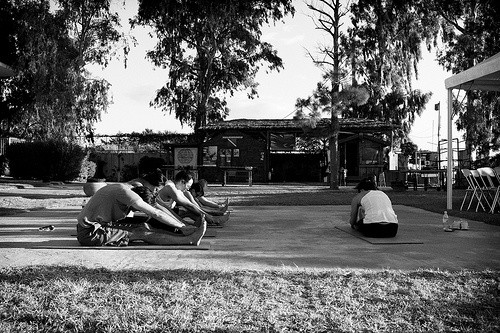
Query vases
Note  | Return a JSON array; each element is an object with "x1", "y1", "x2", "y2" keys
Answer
[{"x1": 83, "y1": 177, "x2": 107, "y2": 197}]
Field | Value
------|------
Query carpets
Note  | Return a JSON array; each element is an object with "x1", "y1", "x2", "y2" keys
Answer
[
  {"x1": 31, "y1": 211, "x2": 231, "y2": 249},
  {"x1": 335, "y1": 224, "x2": 424, "y2": 244}
]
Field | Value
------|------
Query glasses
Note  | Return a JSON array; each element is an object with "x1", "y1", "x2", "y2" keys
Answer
[{"x1": 195, "y1": 193, "x2": 199, "y2": 198}]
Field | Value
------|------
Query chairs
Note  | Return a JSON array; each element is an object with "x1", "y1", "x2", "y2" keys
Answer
[{"x1": 460, "y1": 166, "x2": 500, "y2": 214}]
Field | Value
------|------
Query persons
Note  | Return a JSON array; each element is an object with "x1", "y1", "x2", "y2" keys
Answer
[
  {"x1": 157, "y1": 171, "x2": 230, "y2": 227},
  {"x1": 113, "y1": 168, "x2": 180, "y2": 231},
  {"x1": 77, "y1": 184, "x2": 207, "y2": 246},
  {"x1": 350, "y1": 182, "x2": 399, "y2": 238}
]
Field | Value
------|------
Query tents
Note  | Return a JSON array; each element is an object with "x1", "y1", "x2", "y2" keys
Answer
[{"x1": 445, "y1": 52, "x2": 500, "y2": 210}]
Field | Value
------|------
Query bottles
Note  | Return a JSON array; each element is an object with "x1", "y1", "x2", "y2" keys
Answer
[{"x1": 442, "y1": 211, "x2": 449, "y2": 230}]
